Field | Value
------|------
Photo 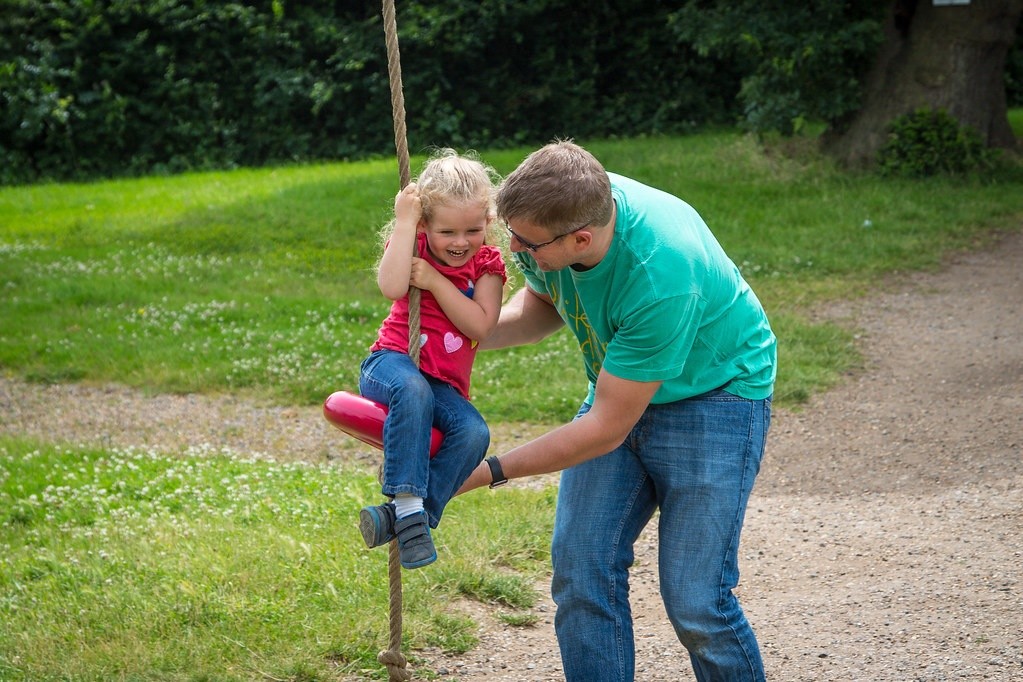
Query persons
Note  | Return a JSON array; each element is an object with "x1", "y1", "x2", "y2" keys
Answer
[
  {"x1": 450, "y1": 134, "x2": 777, "y2": 682},
  {"x1": 359, "y1": 148, "x2": 527, "y2": 571}
]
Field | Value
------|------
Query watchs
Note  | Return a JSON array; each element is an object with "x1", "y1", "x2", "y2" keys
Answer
[{"x1": 484, "y1": 455, "x2": 508, "y2": 489}]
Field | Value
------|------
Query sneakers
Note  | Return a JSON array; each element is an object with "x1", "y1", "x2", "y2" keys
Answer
[
  {"x1": 359, "y1": 501, "x2": 397, "y2": 550},
  {"x1": 394, "y1": 512, "x2": 438, "y2": 568}
]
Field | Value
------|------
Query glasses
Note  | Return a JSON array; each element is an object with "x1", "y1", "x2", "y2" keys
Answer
[{"x1": 504, "y1": 218, "x2": 593, "y2": 251}]
